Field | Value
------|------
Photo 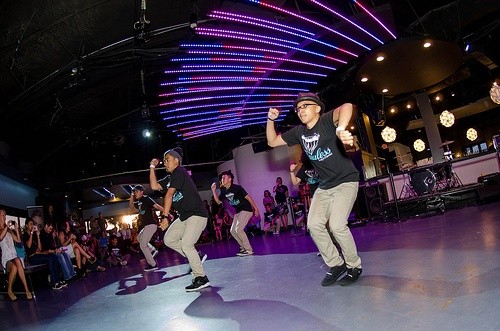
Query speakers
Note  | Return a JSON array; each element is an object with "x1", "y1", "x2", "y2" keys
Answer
[
  {"x1": 475, "y1": 184, "x2": 500, "y2": 205},
  {"x1": 355, "y1": 183, "x2": 390, "y2": 221}
]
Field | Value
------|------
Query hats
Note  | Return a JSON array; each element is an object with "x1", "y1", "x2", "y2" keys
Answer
[
  {"x1": 294, "y1": 92, "x2": 325, "y2": 115},
  {"x1": 221, "y1": 169, "x2": 234, "y2": 179}
]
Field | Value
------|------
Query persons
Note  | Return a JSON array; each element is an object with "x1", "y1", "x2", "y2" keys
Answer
[
  {"x1": 38, "y1": 221, "x2": 78, "y2": 282},
  {"x1": 203, "y1": 195, "x2": 232, "y2": 240},
  {"x1": 290, "y1": 147, "x2": 341, "y2": 256},
  {"x1": 262, "y1": 178, "x2": 309, "y2": 235},
  {"x1": 266, "y1": 92, "x2": 363, "y2": 285},
  {"x1": 80, "y1": 235, "x2": 106, "y2": 272},
  {"x1": 46, "y1": 205, "x2": 58, "y2": 230},
  {"x1": 68, "y1": 211, "x2": 139, "y2": 266},
  {"x1": 58, "y1": 221, "x2": 96, "y2": 278},
  {"x1": 0, "y1": 210, "x2": 33, "y2": 300},
  {"x1": 130, "y1": 185, "x2": 174, "y2": 271},
  {"x1": 22, "y1": 218, "x2": 68, "y2": 290},
  {"x1": 212, "y1": 172, "x2": 259, "y2": 256},
  {"x1": 150, "y1": 151, "x2": 209, "y2": 291}
]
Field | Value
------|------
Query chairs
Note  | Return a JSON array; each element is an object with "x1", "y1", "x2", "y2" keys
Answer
[{"x1": 0, "y1": 262, "x2": 50, "y2": 298}]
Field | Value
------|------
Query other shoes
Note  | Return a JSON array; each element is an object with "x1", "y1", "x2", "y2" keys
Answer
[
  {"x1": 273, "y1": 231, "x2": 279, "y2": 234},
  {"x1": 236, "y1": 249, "x2": 254, "y2": 257},
  {"x1": 190, "y1": 251, "x2": 207, "y2": 273},
  {"x1": 185, "y1": 275, "x2": 210, "y2": 292},
  {"x1": 321, "y1": 262, "x2": 347, "y2": 286},
  {"x1": 338, "y1": 268, "x2": 362, "y2": 286},
  {"x1": 120, "y1": 260, "x2": 127, "y2": 265},
  {"x1": 96, "y1": 266, "x2": 105, "y2": 272},
  {"x1": 144, "y1": 265, "x2": 159, "y2": 272},
  {"x1": 151, "y1": 249, "x2": 158, "y2": 257},
  {"x1": 52, "y1": 281, "x2": 68, "y2": 289}
]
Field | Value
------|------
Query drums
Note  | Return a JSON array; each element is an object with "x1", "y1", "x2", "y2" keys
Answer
[
  {"x1": 409, "y1": 166, "x2": 437, "y2": 195},
  {"x1": 432, "y1": 161, "x2": 453, "y2": 182}
]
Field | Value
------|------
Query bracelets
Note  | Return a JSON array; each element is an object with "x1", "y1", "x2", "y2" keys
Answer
[
  {"x1": 336, "y1": 127, "x2": 345, "y2": 138},
  {"x1": 267, "y1": 116, "x2": 275, "y2": 121},
  {"x1": 161, "y1": 215, "x2": 168, "y2": 219},
  {"x1": 31, "y1": 232, "x2": 33, "y2": 234},
  {"x1": 151, "y1": 165, "x2": 155, "y2": 169}
]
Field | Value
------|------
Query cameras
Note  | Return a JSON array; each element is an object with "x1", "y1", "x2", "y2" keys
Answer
[{"x1": 11, "y1": 221, "x2": 15, "y2": 225}]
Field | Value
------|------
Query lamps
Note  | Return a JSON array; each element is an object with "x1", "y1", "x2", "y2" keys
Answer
[
  {"x1": 466, "y1": 128, "x2": 478, "y2": 140},
  {"x1": 381, "y1": 125, "x2": 397, "y2": 143},
  {"x1": 489, "y1": 82, "x2": 500, "y2": 105},
  {"x1": 413, "y1": 139, "x2": 426, "y2": 152},
  {"x1": 439, "y1": 110, "x2": 455, "y2": 127}
]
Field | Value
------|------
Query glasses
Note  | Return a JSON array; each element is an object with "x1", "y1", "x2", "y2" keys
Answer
[{"x1": 294, "y1": 104, "x2": 317, "y2": 112}]
